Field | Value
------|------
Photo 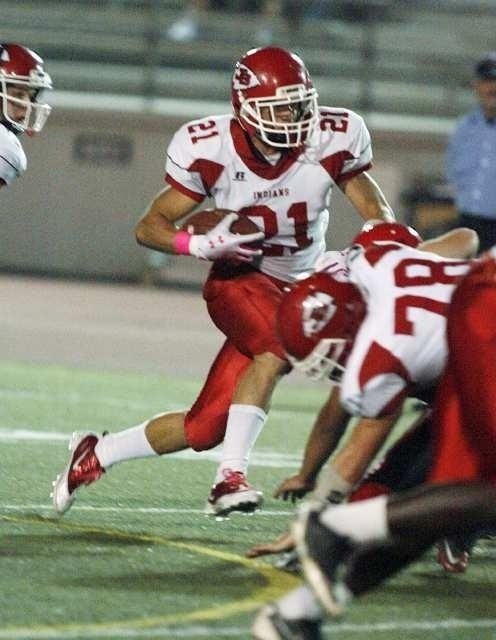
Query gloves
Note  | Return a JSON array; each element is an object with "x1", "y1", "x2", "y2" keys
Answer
[{"x1": 189, "y1": 212, "x2": 265, "y2": 266}]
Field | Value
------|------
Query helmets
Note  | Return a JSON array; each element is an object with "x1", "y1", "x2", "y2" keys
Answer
[
  {"x1": 279, "y1": 271, "x2": 366, "y2": 385},
  {"x1": 0, "y1": 43, "x2": 52, "y2": 136},
  {"x1": 232, "y1": 45, "x2": 320, "y2": 148}
]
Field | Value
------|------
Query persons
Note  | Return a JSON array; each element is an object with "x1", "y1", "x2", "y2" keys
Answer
[
  {"x1": 0, "y1": 43, "x2": 52, "y2": 189},
  {"x1": 51, "y1": 47, "x2": 396, "y2": 515},
  {"x1": 444, "y1": 57, "x2": 496, "y2": 256},
  {"x1": 244, "y1": 222, "x2": 496, "y2": 640}
]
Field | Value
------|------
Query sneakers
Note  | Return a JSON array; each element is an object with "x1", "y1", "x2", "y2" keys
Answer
[
  {"x1": 49, "y1": 431, "x2": 105, "y2": 515},
  {"x1": 253, "y1": 503, "x2": 359, "y2": 640},
  {"x1": 207, "y1": 468, "x2": 262, "y2": 516}
]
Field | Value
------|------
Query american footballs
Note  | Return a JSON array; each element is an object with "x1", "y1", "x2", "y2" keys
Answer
[{"x1": 179, "y1": 209, "x2": 263, "y2": 263}]
